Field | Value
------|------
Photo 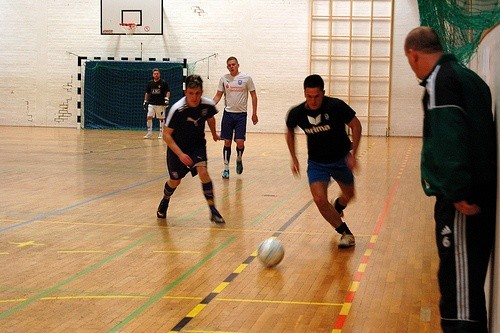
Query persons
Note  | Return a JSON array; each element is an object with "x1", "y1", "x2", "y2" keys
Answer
[
  {"x1": 143, "y1": 68, "x2": 169, "y2": 139},
  {"x1": 208, "y1": 57, "x2": 258, "y2": 179},
  {"x1": 157, "y1": 75, "x2": 225, "y2": 224},
  {"x1": 404, "y1": 26, "x2": 496, "y2": 333},
  {"x1": 286, "y1": 75, "x2": 362, "y2": 249}
]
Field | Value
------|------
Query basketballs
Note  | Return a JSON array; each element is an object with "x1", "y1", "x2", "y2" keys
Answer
[{"x1": 257, "y1": 238, "x2": 285, "y2": 267}]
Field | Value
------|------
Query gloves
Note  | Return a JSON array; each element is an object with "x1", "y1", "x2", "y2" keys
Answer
[
  {"x1": 143, "y1": 100, "x2": 147, "y2": 110},
  {"x1": 164, "y1": 97, "x2": 169, "y2": 109}
]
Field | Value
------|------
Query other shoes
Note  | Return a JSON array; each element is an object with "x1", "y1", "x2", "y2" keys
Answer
[
  {"x1": 159, "y1": 133, "x2": 163, "y2": 138},
  {"x1": 144, "y1": 132, "x2": 152, "y2": 139}
]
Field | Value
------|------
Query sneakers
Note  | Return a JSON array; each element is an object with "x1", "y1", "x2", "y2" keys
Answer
[
  {"x1": 338, "y1": 232, "x2": 355, "y2": 247},
  {"x1": 157, "y1": 197, "x2": 169, "y2": 218},
  {"x1": 211, "y1": 214, "x2": 224, "y2": 223},
  {"x1": 333, "y1": 199, "x2": 343, "y2": 217},
  {"x1": 237, "y1": 160, "x2": 243, "y2": 174},
  {"x1": 222, "y1": 170, "x2": 229, "y2": 177}
]
